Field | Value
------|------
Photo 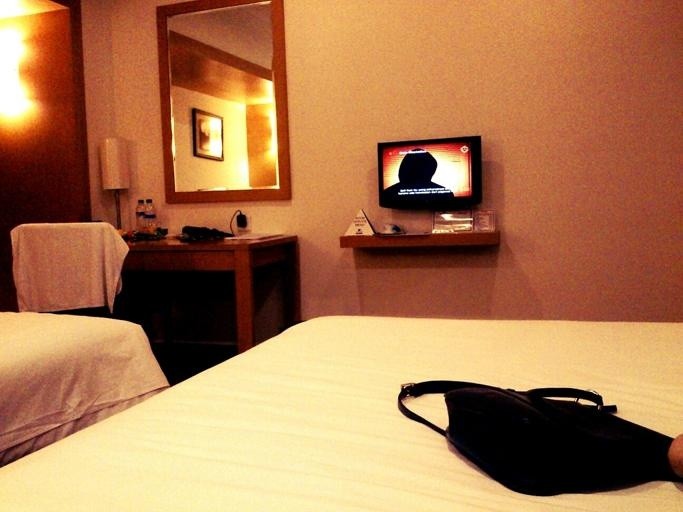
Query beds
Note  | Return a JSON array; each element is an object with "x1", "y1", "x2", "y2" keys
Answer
[
  {"x1": 0, "y1": 311, "x2": 166, "y2": 466},
  {"x1": 0, "y1": 315, "x2": 680, "y2": 512}
]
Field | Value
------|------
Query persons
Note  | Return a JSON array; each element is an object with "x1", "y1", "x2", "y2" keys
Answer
[
  {"x1": 667, "y1": 433, "x2": 683, "y2": 478},
  {"x1": 383, "y1": 145, "x2": 457, "y2": 198}
]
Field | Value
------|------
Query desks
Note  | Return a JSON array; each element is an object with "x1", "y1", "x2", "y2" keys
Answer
[{"x1": 120, "y1": 234, "x2": 298, "y2": 354}]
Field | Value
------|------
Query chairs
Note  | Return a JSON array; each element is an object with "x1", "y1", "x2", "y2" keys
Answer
[{"x1": 11, "y1": 221, "x2": 129, "y2": 321}]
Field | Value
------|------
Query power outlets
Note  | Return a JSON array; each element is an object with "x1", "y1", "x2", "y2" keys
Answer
[{"x1": 234, "y1": 213, "x2": 252, "y2": 233}]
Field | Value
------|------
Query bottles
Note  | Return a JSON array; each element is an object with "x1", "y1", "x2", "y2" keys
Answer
[
  {"x1": 143, "y1": 198, "x2": 156, "y2": 231},
  {"x1": 135, "y1": 198, "x2": 144, "y2": 231}
]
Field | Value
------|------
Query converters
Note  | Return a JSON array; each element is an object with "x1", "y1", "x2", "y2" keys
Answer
[{"x1": 237, "y1": 214, "x2": 246, "y2": 227}]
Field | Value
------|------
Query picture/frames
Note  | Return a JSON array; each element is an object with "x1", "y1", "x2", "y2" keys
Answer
[{"x1": 190, "y1": 107, "x2": 224, "y2": 161}]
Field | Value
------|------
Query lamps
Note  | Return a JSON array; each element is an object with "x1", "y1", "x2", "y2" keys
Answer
[{"x1": 98, "y1": 137, "x2": 134, "y2": 238}]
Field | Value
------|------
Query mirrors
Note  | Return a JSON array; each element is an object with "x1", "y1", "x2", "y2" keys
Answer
[{"x1": 155, "y1": 0, "x2": 293, "y2": 205}]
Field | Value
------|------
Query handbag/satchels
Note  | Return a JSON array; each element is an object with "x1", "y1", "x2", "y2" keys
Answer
[{"x1": 445, "y1": 386, "x2": 681, "y2": 495}]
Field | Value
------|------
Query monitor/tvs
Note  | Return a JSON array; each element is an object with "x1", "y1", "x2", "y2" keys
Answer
[{"x1": 378, "y1": 135, "x2": 482, "y2": 212}]
